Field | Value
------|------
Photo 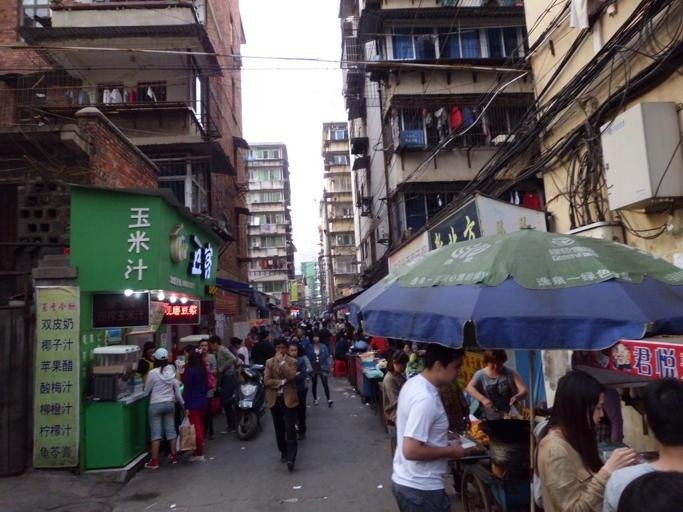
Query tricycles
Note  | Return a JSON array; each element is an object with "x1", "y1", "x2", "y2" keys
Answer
[{"x1": 441, "y1": 418, "x2": 538, "y2": 511}]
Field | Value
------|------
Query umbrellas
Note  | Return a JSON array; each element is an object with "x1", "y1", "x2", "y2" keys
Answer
[{"x1": 347, "y1": 227, "x2": 683, "y2": 470}]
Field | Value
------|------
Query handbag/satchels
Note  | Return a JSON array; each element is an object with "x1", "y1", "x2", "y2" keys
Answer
[{"x1": 179, "y1": 424, "x2": 197, "y2": 451}]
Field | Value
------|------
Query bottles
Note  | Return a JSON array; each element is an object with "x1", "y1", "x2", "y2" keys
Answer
[{"x1": 134, "y1": 374, "x2": 144, "y2": 393}]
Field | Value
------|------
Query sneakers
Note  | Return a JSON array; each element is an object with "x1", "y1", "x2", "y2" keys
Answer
[
  {"x1": 287, "y1": 461, "x2": 294, "y2": 472},
  {"x1": 280, "y1": 456, "x2": 288, "y2": 463},
  {"x1": 327, "y1": 400, "x2": 334, "y2": 407},
  {"x1": 188, "y1": 456, "x2": 204, "y2": 462},
  {"x1": 313, "y1": 400, "x2": 319, "y2": 405},
  {"x1": 168, "y1": 453, "x2": 179, "y2": 464},
  {"x1": 144, "y1": 459, "x2": 160, "y2": 469}
]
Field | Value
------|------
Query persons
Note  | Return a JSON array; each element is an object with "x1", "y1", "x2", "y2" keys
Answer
[
  {"x1": 404, "y1": 341, "x2": 427, "y2": 379},
  {"x1": 572, "y1": 350, "x2": 623, "y2": 444},
  {"x1": 383, "y1": 351, "x2": 409, "y2": 438},
  {"x1": 536, "y1": 370, "x2": 637, "y2": 512},
  {"x1": 369, "y1": 335, "x2": 390, "y2": 355},
  {"x1": 391, "y1": 344, "x2": 465, "y2": 512},
  {"x1": 602, "y1": 376, "x2": 683, "y2": 512},
  {"x1": 138, "y1": 316, "x2": 353, "y2": 471},
  {"x1": 465, "y1": 350, "x2": 528, "y2": 421},
  {"x1": 617, "y1": 470, "x2": 683, "y2": 512}
]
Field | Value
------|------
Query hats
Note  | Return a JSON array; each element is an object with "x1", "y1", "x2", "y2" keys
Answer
[{"x1": 152, "y1": 347, "x2": 169, "y2": 361}]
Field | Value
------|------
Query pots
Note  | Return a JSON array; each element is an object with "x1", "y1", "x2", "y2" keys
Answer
[{"x1": 478, "y1": 418, "x2": 539, "y2": 446}]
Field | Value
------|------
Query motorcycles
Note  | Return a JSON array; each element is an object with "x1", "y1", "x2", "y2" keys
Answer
[{"x1": 235, "y1": 354, "x2": 264, "y2": 438}]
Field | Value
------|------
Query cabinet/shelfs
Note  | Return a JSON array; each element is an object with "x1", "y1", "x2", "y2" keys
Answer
[{"x1": 355, "y1": 356, "x2": 383, "y2": 401}]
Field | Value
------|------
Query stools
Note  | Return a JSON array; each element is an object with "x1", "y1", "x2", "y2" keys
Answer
[{"x1": 333, "y1": 360, "x2": 347, "y2": 378}]
